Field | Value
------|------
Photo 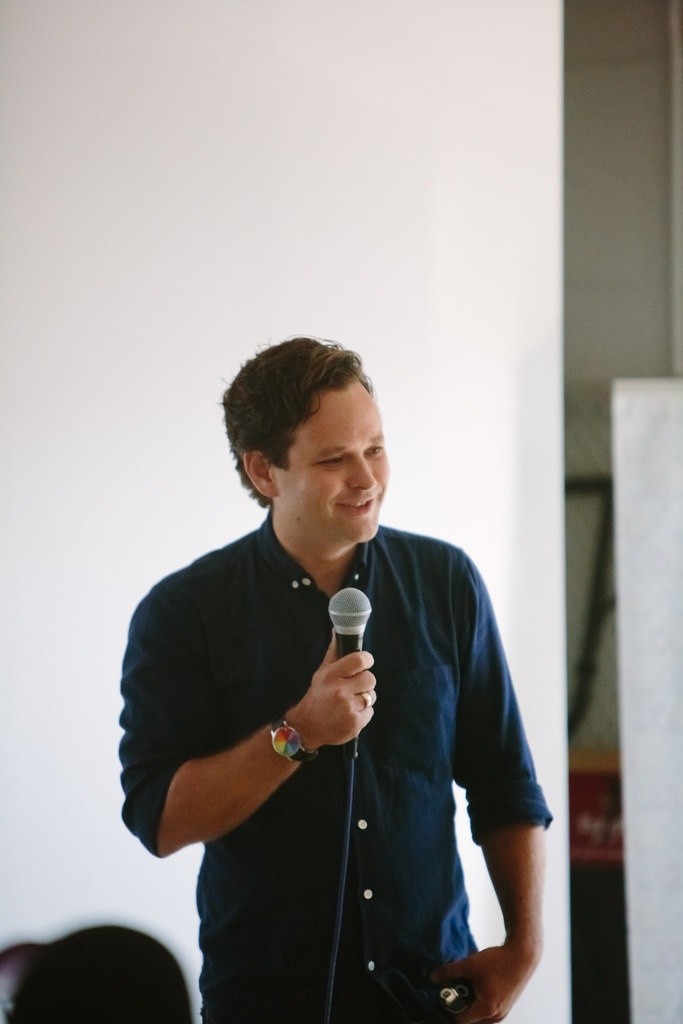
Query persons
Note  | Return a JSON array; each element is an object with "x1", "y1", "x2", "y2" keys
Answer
[
  {"x1": 0, "y1": 924, "x2": 193, "y2": 1024},
  {"x1": 117, "y1": 336, "x2": 556, "y2": 1024}
]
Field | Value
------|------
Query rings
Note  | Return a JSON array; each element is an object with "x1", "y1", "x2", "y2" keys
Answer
[{"x1": 359, "y1": 692, "x2": 373, "y2": 709}]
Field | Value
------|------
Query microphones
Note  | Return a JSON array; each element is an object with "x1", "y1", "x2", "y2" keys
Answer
[{"x1": 328, "y1": 587, "x2": 370, "y2": 759}]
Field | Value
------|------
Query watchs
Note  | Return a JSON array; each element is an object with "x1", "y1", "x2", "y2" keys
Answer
[{"x1": 269, "y1": 711, "x2": 318, "y2": 763}]
